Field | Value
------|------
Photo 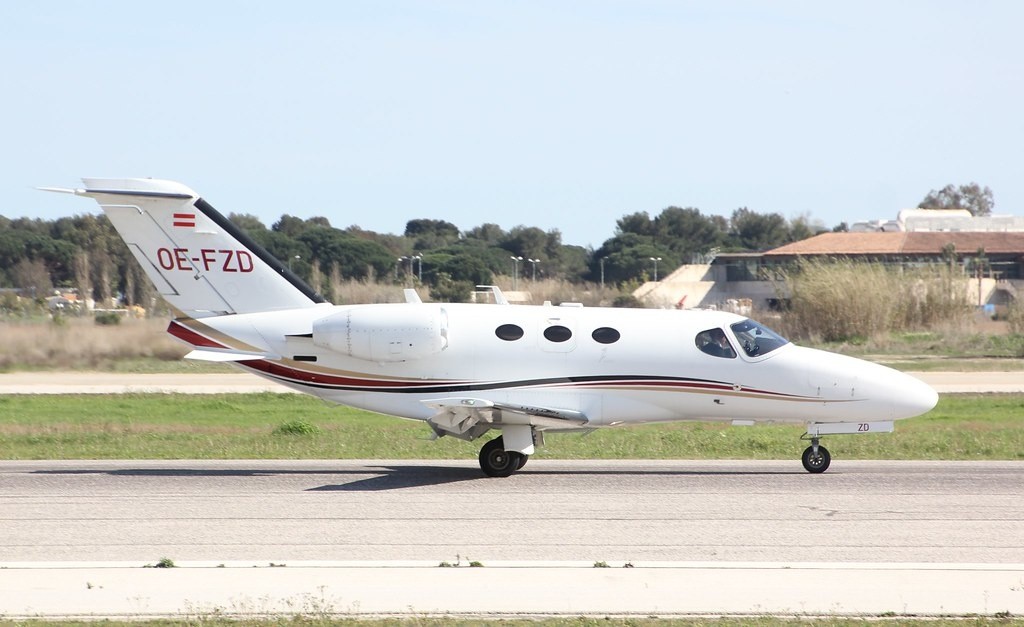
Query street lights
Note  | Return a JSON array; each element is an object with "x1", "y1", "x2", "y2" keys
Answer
[
  {"x1": 510, "y1": 256, "x2": 524, "y2": 291},
  {"x1": 289, "y1": 254, "x2": 301, "y2": 273},
  {"x1": 650, "y1": 257, "x2": 662, "y2": 282},
  {"x1": 601, "y1": 256, "x2": 609, "y2": 285},
  {"x1": 395, "y1": 255, "x2": 423, "y2": 286},
  {"x1": 528, "y1": 258, "x2": 542, "y2": 285}
]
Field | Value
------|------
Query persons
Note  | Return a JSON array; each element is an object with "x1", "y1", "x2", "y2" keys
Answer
[{"x1": 702, "y1": 328, "x2": 728, "y2": 357}]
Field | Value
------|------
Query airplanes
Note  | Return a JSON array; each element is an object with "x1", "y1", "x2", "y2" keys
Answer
[{"x1": 33, "y1": 176, "x2": 940, "y2": 479}]
